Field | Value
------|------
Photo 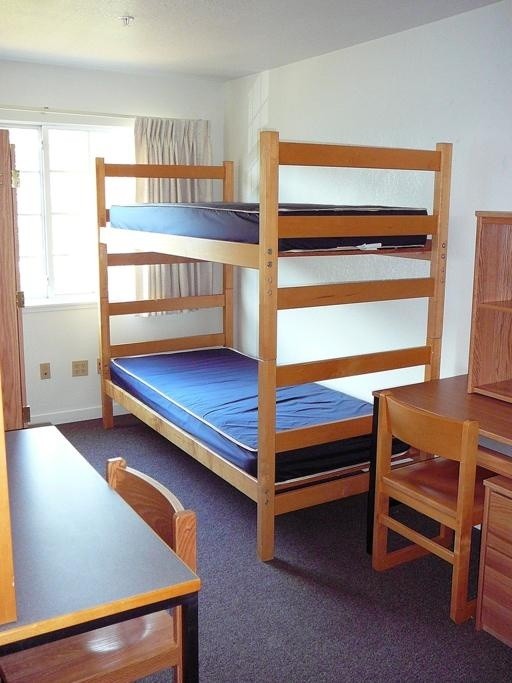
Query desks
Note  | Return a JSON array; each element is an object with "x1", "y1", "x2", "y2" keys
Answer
[{"x1": 365, "y1": 374, "x2": 512, "y2": 649}]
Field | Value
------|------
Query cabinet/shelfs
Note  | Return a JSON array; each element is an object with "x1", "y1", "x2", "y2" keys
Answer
[{"x1": 466, "y1": 210, "x2": 511, "y2": 402}]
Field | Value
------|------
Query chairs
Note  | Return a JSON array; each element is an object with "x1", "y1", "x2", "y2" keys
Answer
[{"x1": 371, "y1": 389, "x2": 497, "y2": 625}]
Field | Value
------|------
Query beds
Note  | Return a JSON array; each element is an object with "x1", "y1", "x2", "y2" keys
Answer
[{"x1": 93, "y1": 129, "x2": 453, "y2": 560}]
